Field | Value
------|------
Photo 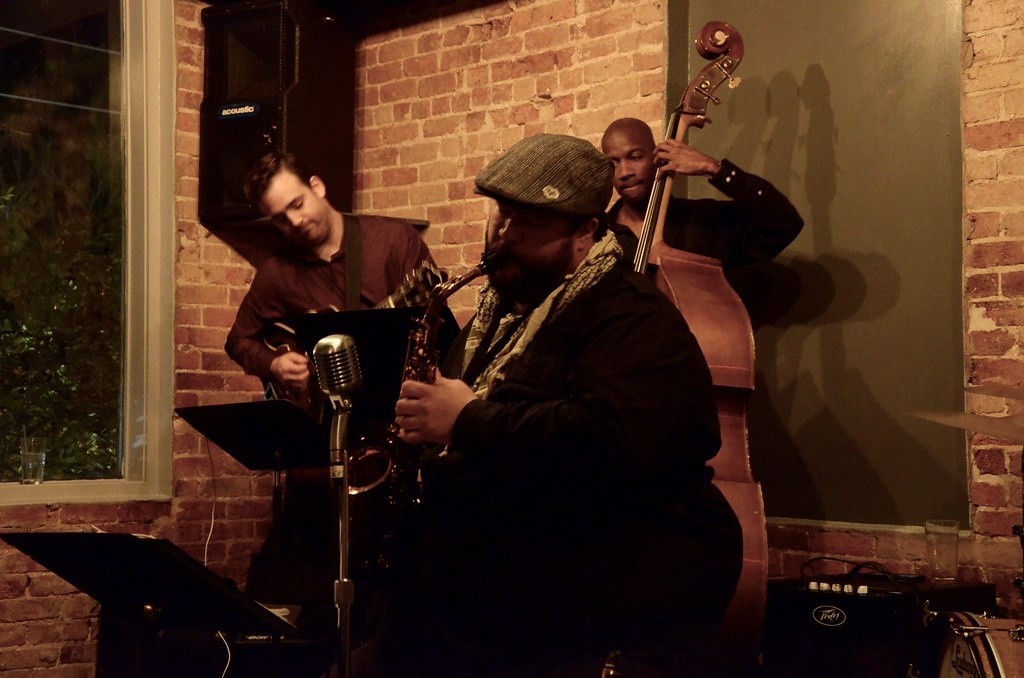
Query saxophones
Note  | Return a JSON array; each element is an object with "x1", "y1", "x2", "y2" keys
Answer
[{"x1": 347, "y1": 249, "x2": 509, "y2": 574}]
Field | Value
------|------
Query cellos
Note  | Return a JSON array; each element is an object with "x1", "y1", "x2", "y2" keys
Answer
[{"x1": 631, "y1": 21, "x2": 769, "y2": 678}]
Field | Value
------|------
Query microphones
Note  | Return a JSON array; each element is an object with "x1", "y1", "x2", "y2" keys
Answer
[{"x1": 313, "y1": 334, "x2": 364, "y2": 413}]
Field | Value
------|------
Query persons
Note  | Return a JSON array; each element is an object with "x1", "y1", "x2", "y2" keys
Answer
[
  {"x1": 318, "y1": 132, "x2": 744, "y2": 676},
  {"x1": 602, "y1": 119, "x2": 805, "y2": 280},
  {"x1": 224, "y1": 151, "x2": 448, "y2": 422}
]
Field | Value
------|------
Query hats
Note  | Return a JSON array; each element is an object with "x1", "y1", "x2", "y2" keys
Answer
[{"x1": 472, "y1": 133, "x2": 617, "y2": 223}]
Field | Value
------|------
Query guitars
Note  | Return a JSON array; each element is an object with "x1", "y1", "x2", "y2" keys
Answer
[{"x1": 258, "y1": 259, "x2": 451, "y2": 426}]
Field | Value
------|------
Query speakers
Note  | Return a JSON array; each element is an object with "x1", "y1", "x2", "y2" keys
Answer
[{"x1": 197, "y1": 0, "x2": 356, "y2": 229}]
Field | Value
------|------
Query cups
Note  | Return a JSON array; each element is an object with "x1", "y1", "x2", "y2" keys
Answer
[{"x1": 17, "y1": 434, "x2": 49, "y2": 486}]
